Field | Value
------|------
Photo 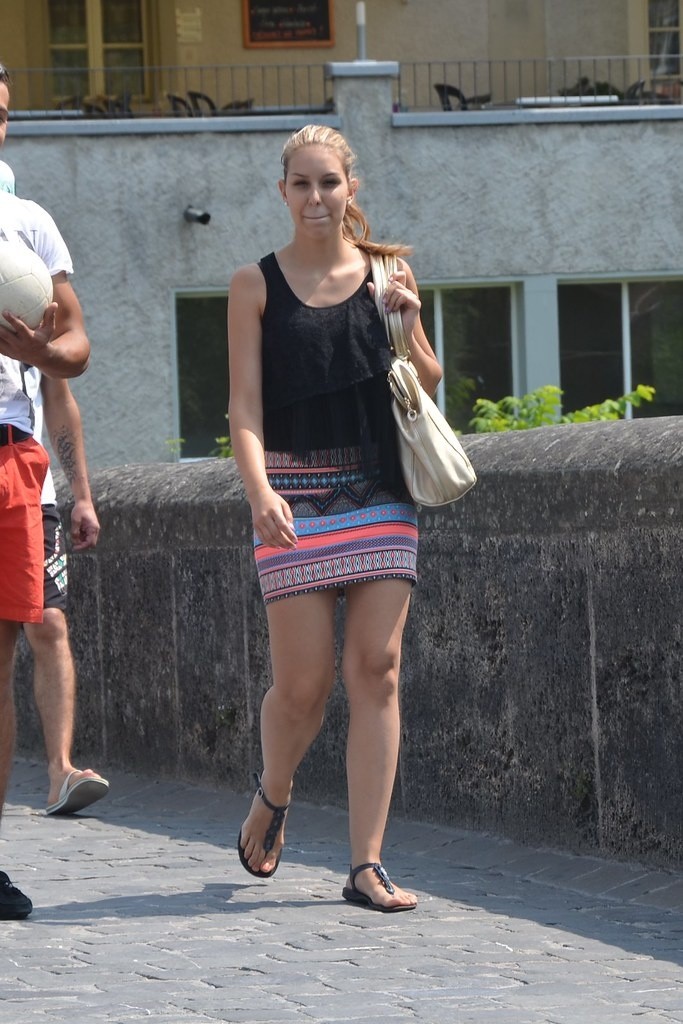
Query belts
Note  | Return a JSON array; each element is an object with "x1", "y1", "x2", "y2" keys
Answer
[{"x1": 0, "y1": 424, "x2": 32, "y2": 447}]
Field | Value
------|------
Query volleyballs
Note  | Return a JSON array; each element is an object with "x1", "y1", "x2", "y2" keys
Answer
[{"x1": 0, "y1": 242, "x2": 54, "y2": 332}]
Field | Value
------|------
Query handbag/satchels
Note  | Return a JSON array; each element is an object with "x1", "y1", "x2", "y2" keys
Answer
[{"x1": 369, "y1": 252, "x2": 476, "y2": 507}]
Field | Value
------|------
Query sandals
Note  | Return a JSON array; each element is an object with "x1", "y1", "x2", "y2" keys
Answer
[
  {"x1": 237, "y1": 768, "x2": 293, "y2": 878},
  {"x1": 342, "y1": 862, "x2": 417, "y2": 911}
]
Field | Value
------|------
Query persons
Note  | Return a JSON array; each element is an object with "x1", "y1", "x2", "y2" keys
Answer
[
  {"x1": 0, "y1": 65, "x2": 112, "y2": 921},
  {"x1": 229, "y1": 125, "x2": 444, "y2": 914}
]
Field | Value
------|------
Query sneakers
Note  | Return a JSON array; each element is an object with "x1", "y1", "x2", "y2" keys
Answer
[{"x1": 0, "y1": 871, "x2": 33, "y2": 920}]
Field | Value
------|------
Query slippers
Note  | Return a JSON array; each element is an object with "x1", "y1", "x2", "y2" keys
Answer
[{"x1": 46, "y1": 770, "x2": 109, "y2": 815}]
